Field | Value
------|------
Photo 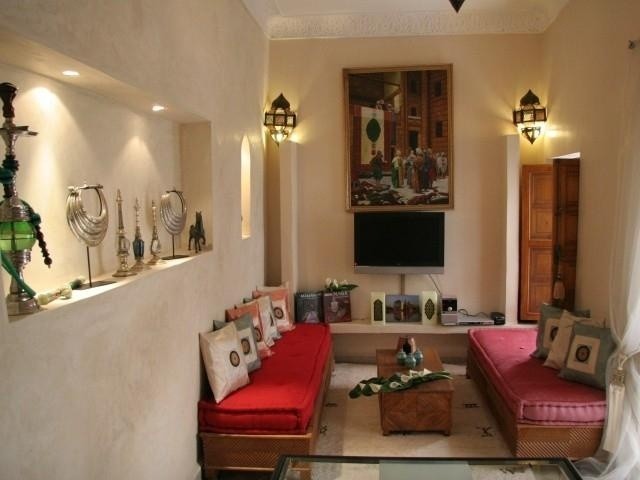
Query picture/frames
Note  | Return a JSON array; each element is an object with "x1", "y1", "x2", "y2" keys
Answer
[{"x1": 341, "y1": 63, "x2": 455, "y2": 214}]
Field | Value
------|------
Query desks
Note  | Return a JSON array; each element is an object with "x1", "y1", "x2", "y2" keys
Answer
[{"x1": 269, "y1": 453, "x2": 586, "y2": 480}]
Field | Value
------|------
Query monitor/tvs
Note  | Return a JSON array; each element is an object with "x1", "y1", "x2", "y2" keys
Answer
[{"x1": 353, "y1": 211, "x2": 445, "y2": 275}]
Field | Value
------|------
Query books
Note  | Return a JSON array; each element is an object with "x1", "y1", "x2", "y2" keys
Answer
[
  {"x1": 321, "y1": 291, "x2": 352, "y2": 324},
  {"x1": 297, "y1": 292, "x2": 321, "y2": 323}
]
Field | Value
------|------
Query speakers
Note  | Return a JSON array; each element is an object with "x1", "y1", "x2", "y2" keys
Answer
[
  {"x1": 439, "y1": 297, "x2": 459, "y2": 326},
  {"x1": 370, "y1": 291, "x2": 386, "y2": 325},
  {"x1": 421, "y1": 290, "x2": 438, "y2": 326}
]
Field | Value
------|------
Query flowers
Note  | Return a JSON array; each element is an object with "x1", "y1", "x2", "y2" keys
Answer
[{"x1": 314, "y1": 277, "x2": 360, "y2": 294}]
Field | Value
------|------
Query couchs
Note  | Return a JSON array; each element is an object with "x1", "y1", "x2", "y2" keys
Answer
[
  {"x1": 465, "y1": 325, "x2": 607, "y2": 460},
  {"x1": 197, "y1": 321, "x2": 336, "y2": 480}
]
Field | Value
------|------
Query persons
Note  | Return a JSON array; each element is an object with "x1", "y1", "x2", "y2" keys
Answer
[{"x1": 367, "y1": 144, "x2": 449, "y2": 192}]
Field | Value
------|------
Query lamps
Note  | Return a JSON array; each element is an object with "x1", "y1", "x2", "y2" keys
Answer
[
  {"x1": 512, "y1": 89, "x2": 548, "y2": 145},
  {"x1": 263, "y1": 92, "x2": 298, "y2": 148}
]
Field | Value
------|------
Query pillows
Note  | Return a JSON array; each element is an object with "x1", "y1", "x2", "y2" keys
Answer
[
  {"x1": 251, "y1": 288, "x2": 297, "y2": 333},
  {"x1": 212, "y1": 311, "x2": 262, "y2": 374},
  {"x1": 242, "y1": 295, "x2": 283, "y2": 341},
  {"x1": 225, "y1": 302, "x2": 276, "y2": 361},
  {"x1": 555, "y1": 321, "x2": 620, "y2": 393},
  {"x1": 198, "y1": 320, "x2": 252, "y2": 405},
  {"x1": 542, "y1": 308, "x2": 608, "y2": 373},
  {"x1": 256, "y1": 281, "x2": 291, "y2": 318},
  {"x1": 556, "y1": 322, "x2": 598, "y2": 390}
]
